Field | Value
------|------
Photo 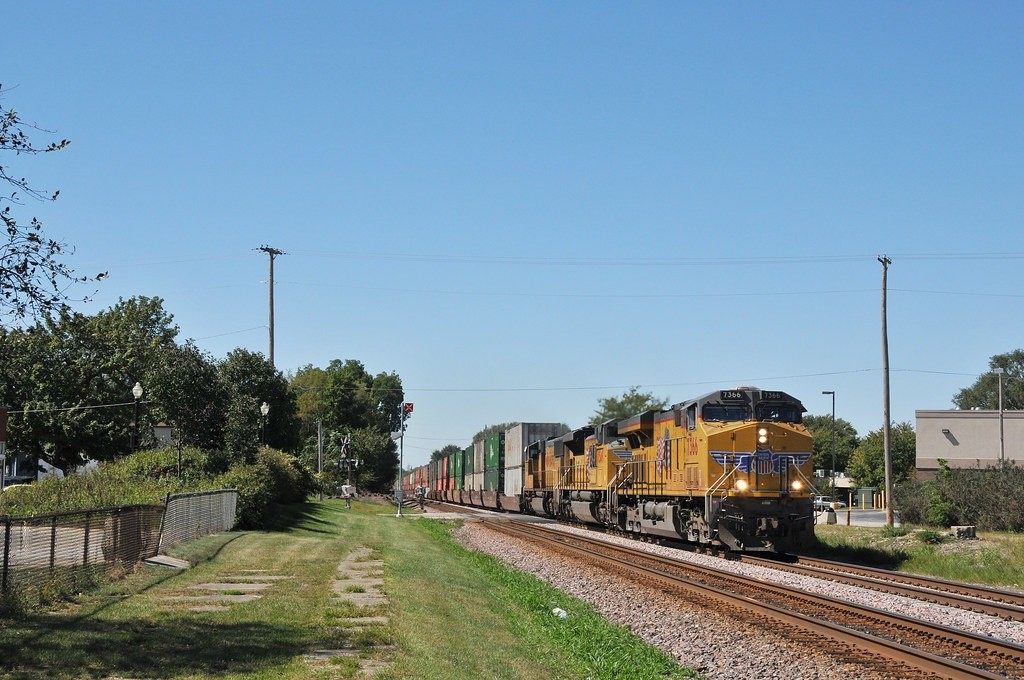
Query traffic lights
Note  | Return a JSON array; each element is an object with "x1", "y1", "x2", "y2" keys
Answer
[{"x1": 405, "y1": 403, "x2": 413, "y2": 412}]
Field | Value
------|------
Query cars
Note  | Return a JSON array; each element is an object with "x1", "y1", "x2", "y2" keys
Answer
[{"x1": 813, "y1": 496, "x2": 846, "y2": 511}]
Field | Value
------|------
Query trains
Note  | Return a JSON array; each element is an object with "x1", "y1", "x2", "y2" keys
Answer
[{"x1": 394, "y1": 386, "x2": 823, "y2": 553}]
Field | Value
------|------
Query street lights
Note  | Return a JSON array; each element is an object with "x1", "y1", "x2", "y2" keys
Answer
[
  {"x1": 133, "y1": 382, "x2": 143, "y2": 452},
  {"x1": 994, "y1": 368, "x2": 1004, "y2": 469},
  {"x1": 260, "y1": 402, "x2": 269, "y2": 446},
  {"x1": 822, "y1": 391, "x2": 835, "y2": 501}
]
